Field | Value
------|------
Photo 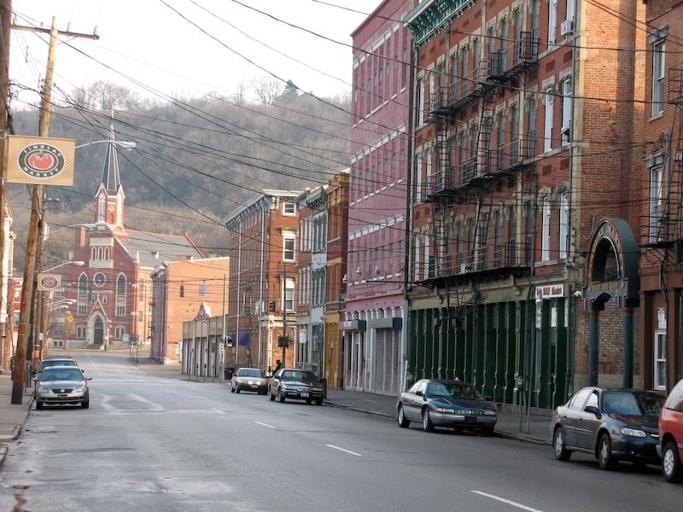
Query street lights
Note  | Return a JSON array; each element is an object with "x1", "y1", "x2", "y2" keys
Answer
[{"x1": 25, "y1": 129, "x2": 138, "y2": 386}]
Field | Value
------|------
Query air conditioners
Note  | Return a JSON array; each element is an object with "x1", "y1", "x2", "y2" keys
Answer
[{"x1": 560, "y1": 20, "x2": 572, "y2": 35}]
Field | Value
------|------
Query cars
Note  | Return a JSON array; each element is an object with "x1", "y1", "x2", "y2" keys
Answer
[
  {"x1": 229, "y1": 366, "x2": 270, "y2": 396},
  {"x1": 394, "y1": 376, "x2": 498, "y2": 438},
  {"x1": 33, "y1": 354, "x2": 92, "y2": 410},
  {"x1": 655, "y1": 374, "x2": 683, "y2": 485},
  {"x1": 548, "y1": 383, "x2": 667, "y2": 471},
  {"x1": 269, "y1": 366, "x2": 327, "y2": 406}
]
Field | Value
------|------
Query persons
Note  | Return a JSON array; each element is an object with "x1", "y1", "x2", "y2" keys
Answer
[
  {"x1": 271, "y1": 359, "x2": 281, "y2": 376},
  {"x1": 9, "y1": 355, "x2": 15, "y2": 380}
]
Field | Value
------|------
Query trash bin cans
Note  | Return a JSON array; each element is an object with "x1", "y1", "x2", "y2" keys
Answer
[{"x1": 224, "y1": 367, "x2": 236, "y2": 380}]
